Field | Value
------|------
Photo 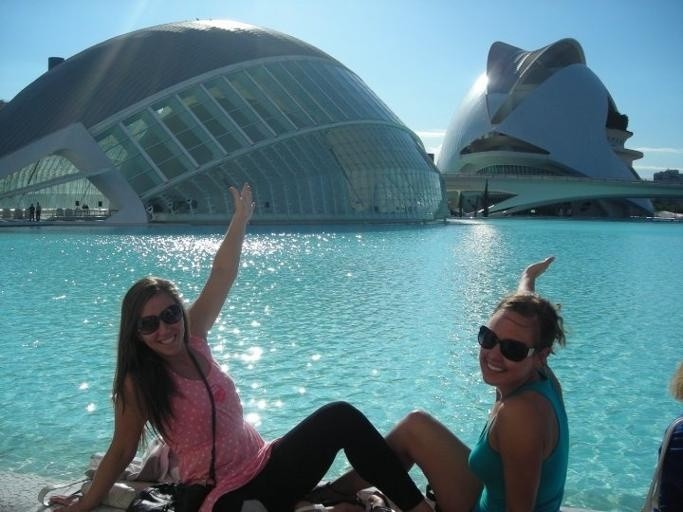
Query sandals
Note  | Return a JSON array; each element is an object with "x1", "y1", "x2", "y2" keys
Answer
[
  {"x1": 368, "y1": 490, "x2": 396, "y2": 512},
  {"x1": 299, "y1": 481, "x2": 360, "y2": 506}
]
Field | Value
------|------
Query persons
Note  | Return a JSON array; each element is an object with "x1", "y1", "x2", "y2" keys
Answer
[
  {"x1": 300, "y1": 254, "x2": 568, "y2": 512},
  {"x1": 25, "y1": 203, "x2": 35, "y2": 222},
  {"x1": 48, "y1": 179, "x2": 437, "y2": 512},
  {"x1": 651, "y1": 362, "x2": 683, "y2": 512},
  {"x1": 35, "y1": 201, "x2": 40, "y2": 221}
]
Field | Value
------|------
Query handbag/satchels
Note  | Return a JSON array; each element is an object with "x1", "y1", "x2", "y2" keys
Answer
[{"x1": 126, "y1": 482, "x2": 219, "y2": 512}]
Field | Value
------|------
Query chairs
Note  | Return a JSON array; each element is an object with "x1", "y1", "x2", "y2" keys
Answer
[{"x1": 0, "y1": 205, "x2": 90, "y2": 219}]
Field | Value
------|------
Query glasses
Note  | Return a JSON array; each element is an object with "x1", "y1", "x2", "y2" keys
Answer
[
  {"x1": 135, "y1": 304, "x2": 185, "y2": 336},
  {"x1": 478, "y1": 324, "x2": 541, "y2": 363}
]
